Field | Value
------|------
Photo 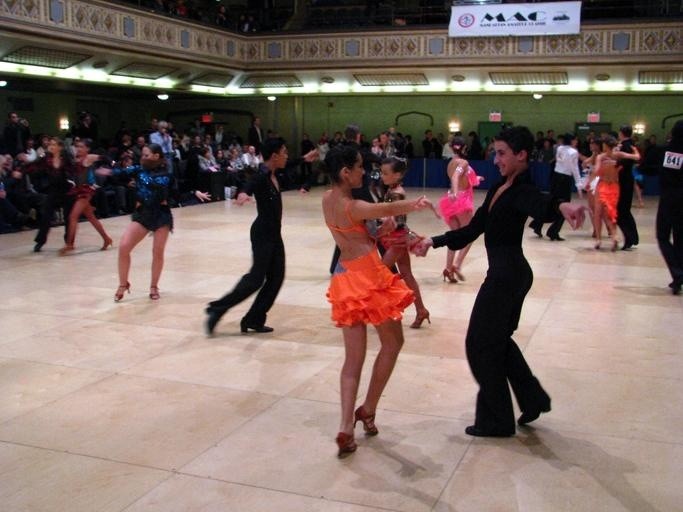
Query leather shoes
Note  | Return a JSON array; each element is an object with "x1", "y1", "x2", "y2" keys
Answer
[
  {"x1": 241, "y1": 320, "x2": 272, "y2": 333},
  {"x1": 208, "y1": 307, "x2": 217, "y2": 333},
  {"x1": 466, "y1": 402, "x2": 551, "y2": 437}
]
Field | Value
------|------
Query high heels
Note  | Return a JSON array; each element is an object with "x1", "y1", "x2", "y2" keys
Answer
[
  {"x1": 443, "y1": 266, "x2": 462, "y2": 282},
  {"x1": 411, "y1": 311, "x2": 430, "y2": 328},
  {"x1": 337, "y1": 406, "x2": 377, "y2": 455},
  {"x1": 101, "y1": 240, "x2": 113, "y2": 251},
  {"x1": 115, "y1": 282, "x2": 130, "y2": 300},
  {"x1": 150, "y1": 286, "x2": 159, "y2": 300}
]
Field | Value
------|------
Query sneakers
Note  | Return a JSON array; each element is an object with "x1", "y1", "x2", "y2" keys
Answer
[
  {"x1": 534, "y1": 229, "x2": 639, "y2": 251},
  {"x1": 668, "y1": 280, "x2": 683, "y2": 294}
]
